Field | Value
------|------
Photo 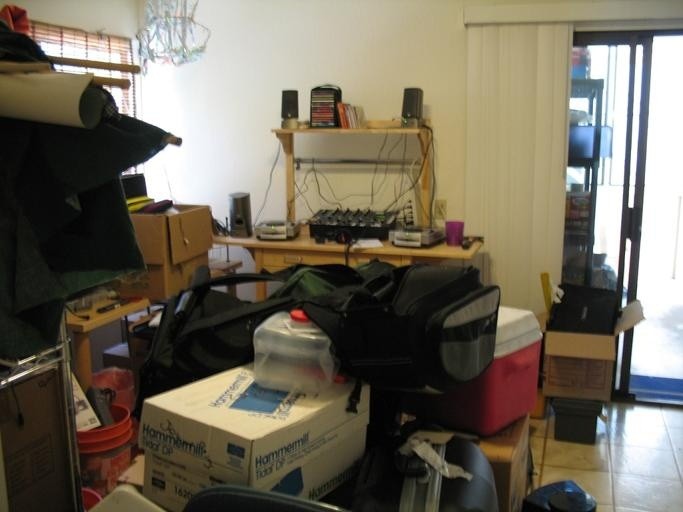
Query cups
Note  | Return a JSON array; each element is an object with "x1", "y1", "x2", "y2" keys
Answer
[{"x1": 446, "y1": 221, "x2": 464, "y2": 246}]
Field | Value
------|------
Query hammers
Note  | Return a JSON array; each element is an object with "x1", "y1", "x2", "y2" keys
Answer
[{"x1": 462, "y1": 236, "x2": 484, "y2": 250}]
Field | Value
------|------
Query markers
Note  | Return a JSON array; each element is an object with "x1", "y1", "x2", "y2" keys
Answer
[{"x1": 97, "y1": 299, "x2": 130, "y2": 313}]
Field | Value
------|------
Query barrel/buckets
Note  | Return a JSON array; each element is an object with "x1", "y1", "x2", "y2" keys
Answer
[{"x1": 76, "y1": 402, "x2": 133, "y2": 498}]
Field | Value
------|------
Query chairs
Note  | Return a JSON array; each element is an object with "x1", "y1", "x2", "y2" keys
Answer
[{"x1": 178, "y1": 486, "x2": 350, "y2": 512}]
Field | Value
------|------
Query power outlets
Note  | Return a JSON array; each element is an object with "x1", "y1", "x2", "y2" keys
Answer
[{"x1": 434, "y1": 200, "x2": 447, "y2": 220}]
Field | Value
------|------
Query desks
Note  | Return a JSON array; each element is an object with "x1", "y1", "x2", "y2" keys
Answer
[
  {"x1": 65, "y1": 257, "x2": 243, "y2": 395},
  {"x1": 214, "y1": 225, "x2": 484, "y2": 300}
]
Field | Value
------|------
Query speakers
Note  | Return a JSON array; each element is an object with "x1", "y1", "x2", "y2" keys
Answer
[
  {"x1": 401, "y1": 87, "x2": 424, "y2": 127},
  {"x1": 280, "y1": 90, "x2": 299, "y2": 120},
  {"x1": 228, "y1": 190, "x2": 252, "y2": 239}
]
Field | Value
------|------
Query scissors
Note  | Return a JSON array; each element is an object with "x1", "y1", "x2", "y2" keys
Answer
[{"x1": 112, "y1": 295, "x2": 143, "y2": 303}]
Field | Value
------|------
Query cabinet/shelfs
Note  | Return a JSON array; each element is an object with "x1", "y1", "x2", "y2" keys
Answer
[
  {"x1": 270, "y1": 118, "x2": 433, "y2": 229},
  {"x1": 561, "y1": 79, "x2": 603, "y2": 286},
  {"x1": 0, "y1": 363, "x2": 77, "y2": 512}
]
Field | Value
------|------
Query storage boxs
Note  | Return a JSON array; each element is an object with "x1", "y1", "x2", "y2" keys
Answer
[
  {"x1": 411, "y1": 411, "x2": 529, "y2": 512},
  {"x1": 543, "y1": 299, "x2": 646, "y2": 402},
  {"x1": 435, "y1": 307, "x2": 543, "y2": 512},
  {"x1": 137, "y1": 359, "x2": 372, "y2": 512},
  {"x1": 105, "y1": 204, "x2": 214, "y2": 300}
]
